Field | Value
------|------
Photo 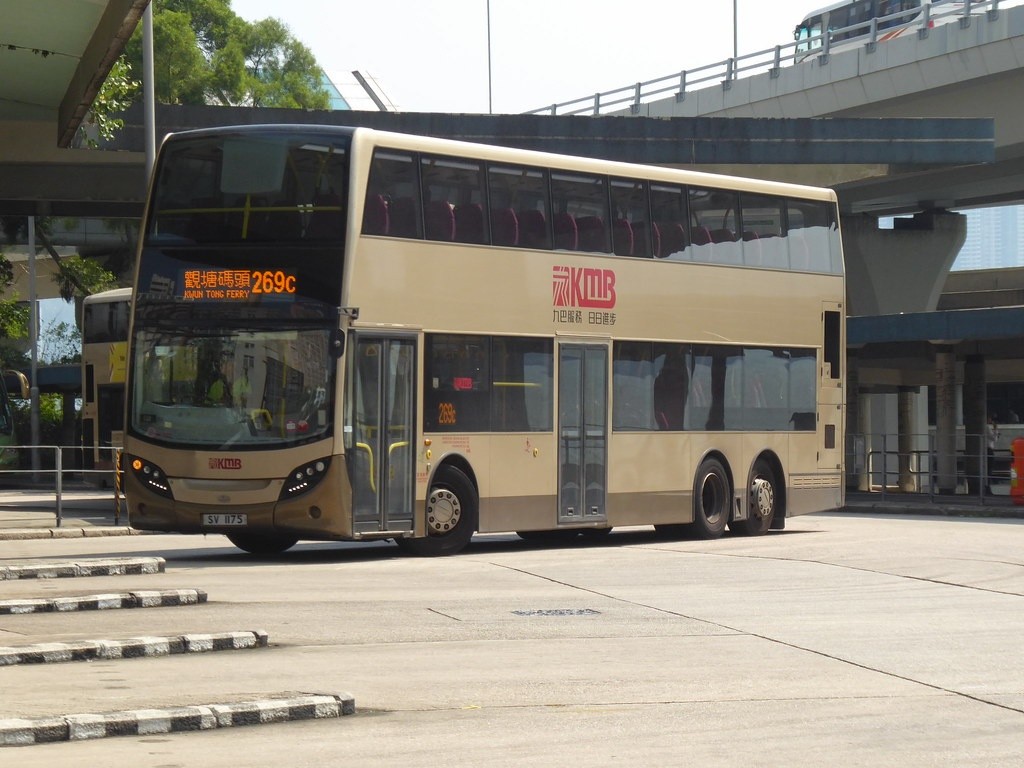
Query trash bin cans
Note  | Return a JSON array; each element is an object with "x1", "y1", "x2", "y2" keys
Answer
[{"x1": 1010, "y1": 440, "x2": 1024, "y2": 505}]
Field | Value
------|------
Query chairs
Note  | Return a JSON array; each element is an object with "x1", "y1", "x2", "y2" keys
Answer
[
  {"x1": 389, "y1": 196, "x2": 421, "y2": 239},
  {"x1": 631, "y1": 221, "x2": 660, "y2": 258},
  {"x1": 658, "y1": 222, "x2": 689, "y2": 258},
  {"x1": 691, "y1": 225, "x2": 714, "y2": 263},
  {"x1": 790, "y1": 235, "x2": 810, "y2": 271},
  {"x1": 181, "y1": 191, "x2": 226, "y2": 240},
  {"x1": 423, "y1": 358, "x2": 490, "y2": 432},
  {"x1": 613, "y1": 219, "x2": 634, "y2": 256},
  {"x1": 574, "y1": 215, "x2": 606, "y2": 252},
  {"x1": 758, "y1": 233, "x2": 783, "y2": 253},
  {"x1": 732, "y1": 231, "x2": 762, "y2": 268},
  {"x1": 452, "y1": 203, "x2": 487, "y2": 245},
  {"x1": 343, "y1": 193, "x2": 390, "y2": 235},
  {"x1": 709, "y1": 229, "x2": 738, "y2": 245},
  {"x1": 491, "y1": 208, "x2": 518, "y2": 246},
  {"x1": 516, "y1": 209, "x2": 547, "y2": 249},
  {"x1": 303, "y1": 199, "x2": 345, "y2": 237},
  {"x1": 553, "y1": 213, "x2": 578, "y2": 251},
  {"x1": 263, "y1": 201, "x2": 305, "y2": 238},
  {"x1": 423, "y1": 201, "x2": 455, "y2": 242}
]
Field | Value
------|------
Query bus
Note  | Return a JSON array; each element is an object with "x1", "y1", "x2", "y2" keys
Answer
[
  {"x1": 118, "y1": 123, "x2": 848, "y2": 558},
  {"x1": 791, "y1": 0, "x2": 989, "y2": 64},
  {"x1": 0, "y1": 369, "x2": 31, "y2": 475},
  {"x1": 79, "y1": 284, "x2": 134, "y2": 493}
]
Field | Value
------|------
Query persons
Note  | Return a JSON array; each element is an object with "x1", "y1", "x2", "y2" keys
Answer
[
  {"x1": 202, "y1": 350, "x2": 252, "y2": 413},
  {"x1": 1008, "y1": 408, "x2": 1021, "y2": 424},
  {"x1": 981, "y1": 410, "x2": 1003, "y2": 495}
]
